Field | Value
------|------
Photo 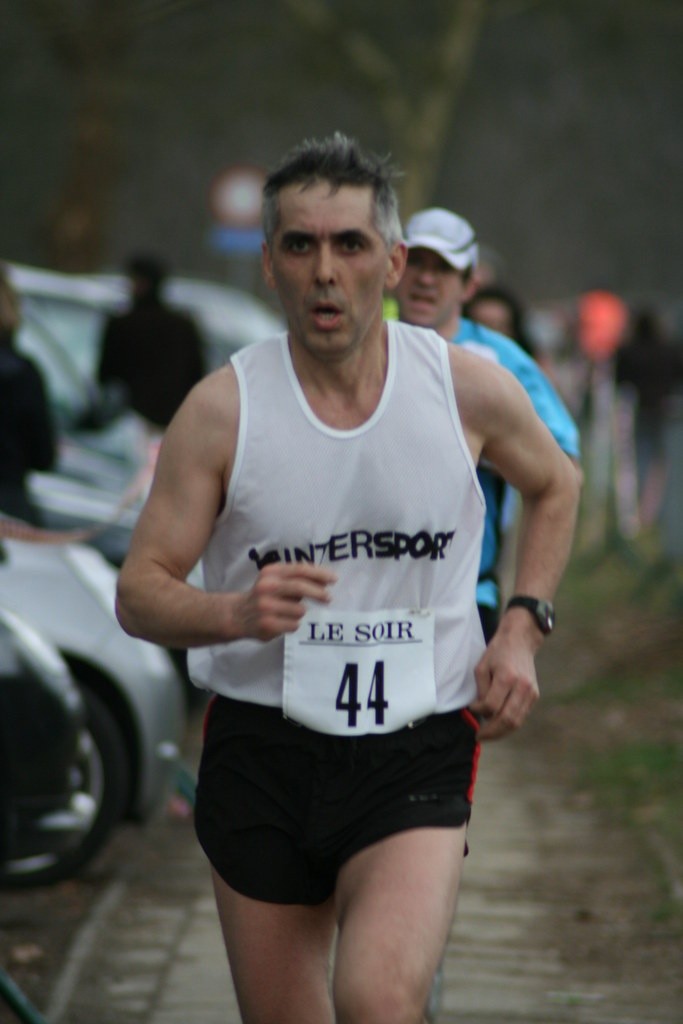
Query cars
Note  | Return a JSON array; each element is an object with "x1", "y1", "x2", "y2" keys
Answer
[{"x1": 1, "y1": 257, "x2": 289, "y2": 889}]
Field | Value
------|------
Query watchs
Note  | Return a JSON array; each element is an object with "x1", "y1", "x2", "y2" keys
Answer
[{"x1": 508, "y1": 594, "x2": 556, "y2": 634}]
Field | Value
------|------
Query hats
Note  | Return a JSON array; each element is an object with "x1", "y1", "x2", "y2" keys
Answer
[{"x1": 402, "y1": 207, "x2": 479, "y2": 270}]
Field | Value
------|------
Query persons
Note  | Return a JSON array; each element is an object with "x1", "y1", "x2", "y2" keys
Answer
[
  {"x1": 0, "y1": 207, "x2": 683, "y2": 720},
  {"x1": 113, "y1": 135, "x2": 582, "y2": 1024}
]
